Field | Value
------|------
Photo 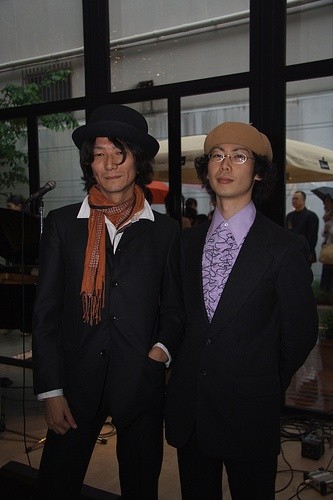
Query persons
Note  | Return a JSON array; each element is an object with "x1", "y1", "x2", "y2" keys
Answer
[
  {"x1": 180, "y1": 191, "x2": 333, "y2": 295},
  {"x1": 165, "y1": 120, "x2": 319, "y2": 500},
  {"x1": 32, "y1": 105, "x2": 186, "y2": 500}
]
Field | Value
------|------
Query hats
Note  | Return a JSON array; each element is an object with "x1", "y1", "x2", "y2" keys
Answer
[
  {"x1": 203, "y1": 121, "x2": 273, "y2": 159},
  {"x1": 71, "y1": 103, "x2": 160, "y2": 158}
]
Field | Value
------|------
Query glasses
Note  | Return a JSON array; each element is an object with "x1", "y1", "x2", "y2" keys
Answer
[{"x1": 209, "y1": 149, "x2": 256, "y2": 165}]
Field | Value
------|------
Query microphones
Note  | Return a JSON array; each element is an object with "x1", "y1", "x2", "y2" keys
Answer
[{"x1": 24, "y1": 181, "x2": 56, "y2": 205}]
